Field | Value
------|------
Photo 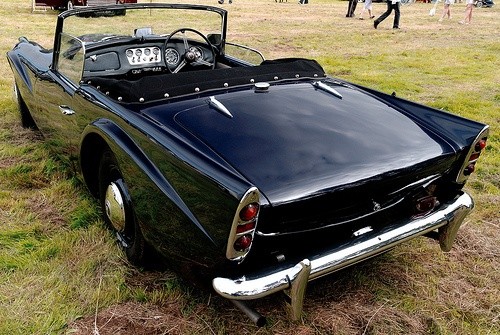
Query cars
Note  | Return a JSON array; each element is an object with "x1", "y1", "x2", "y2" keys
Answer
[{"x1": 6, "y1": 2, "x2": 491, "y2": 328}]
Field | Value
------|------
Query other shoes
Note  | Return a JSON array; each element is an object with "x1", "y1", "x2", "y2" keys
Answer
[
  {"x1": 370, "y1": 16, "x2": 375, "y2": 19},
  {"x1": 393, "y1": 25, "x2": 400, "y2": 29},
  {"x1": 374, "y1": 21, "x2": 377, "y2": 29},
  {"x1": 359, "y1": 18, "x2": 365, "y2": 20}
]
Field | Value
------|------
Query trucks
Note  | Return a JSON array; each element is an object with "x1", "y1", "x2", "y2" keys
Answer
[{"x1": 35, "y1": 0, "x2": 138, "y2": 17}]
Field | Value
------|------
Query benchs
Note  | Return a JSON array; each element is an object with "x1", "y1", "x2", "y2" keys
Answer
[{"x1": 127, "y1": 66, "x2": 265, "y2": 99}]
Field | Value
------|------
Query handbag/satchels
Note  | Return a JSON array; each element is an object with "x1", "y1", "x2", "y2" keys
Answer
[{"x1": 429, "y1": 8, "x2": 435, "y2": 17}]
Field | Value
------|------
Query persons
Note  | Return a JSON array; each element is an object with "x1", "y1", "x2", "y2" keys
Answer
[{"x1": 275, "y1": 0, "x2": 473, "y2": 30}]
